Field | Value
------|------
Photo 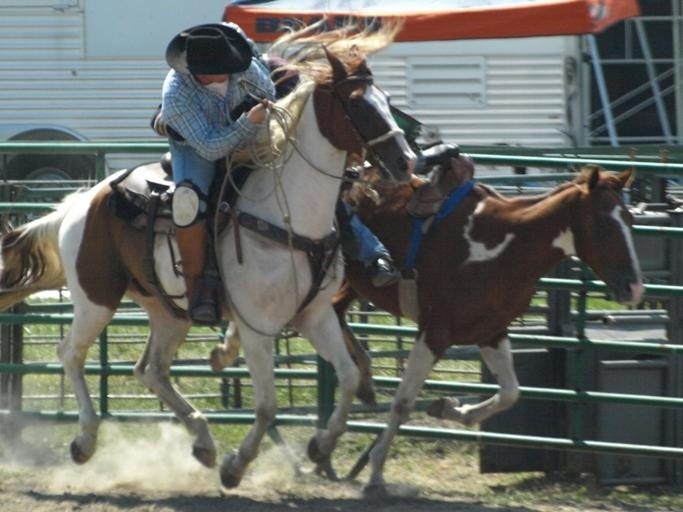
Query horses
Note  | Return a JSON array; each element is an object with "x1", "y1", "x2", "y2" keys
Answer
[
  {"x1": 0, "y1": 45, "x2": 417, "y2": 488},
  {"x1": 210, "y1": 6, "x2": 644, "y2": 497}
]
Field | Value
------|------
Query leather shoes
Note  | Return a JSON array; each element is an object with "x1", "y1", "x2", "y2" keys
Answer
[{"x1": 191, "y1": 285, "x2": 222, "y2": 324}]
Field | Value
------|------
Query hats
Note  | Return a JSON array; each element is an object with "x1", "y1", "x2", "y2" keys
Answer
[{"x1": 165, "y1": 21, "x2": 253, "y2": 78}]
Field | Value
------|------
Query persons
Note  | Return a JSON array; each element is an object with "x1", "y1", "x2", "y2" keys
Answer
[
  {"x1": 337, "y1": 195, "x2": 403, "y2": 289},
  {"x1": 151, "y1": 19, "x2": 278, "y2": 327}
]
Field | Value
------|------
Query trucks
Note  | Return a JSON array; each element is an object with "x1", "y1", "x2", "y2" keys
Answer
[{"x1": 0, "y1": 0, "x2": 592, "y2": 263}]
